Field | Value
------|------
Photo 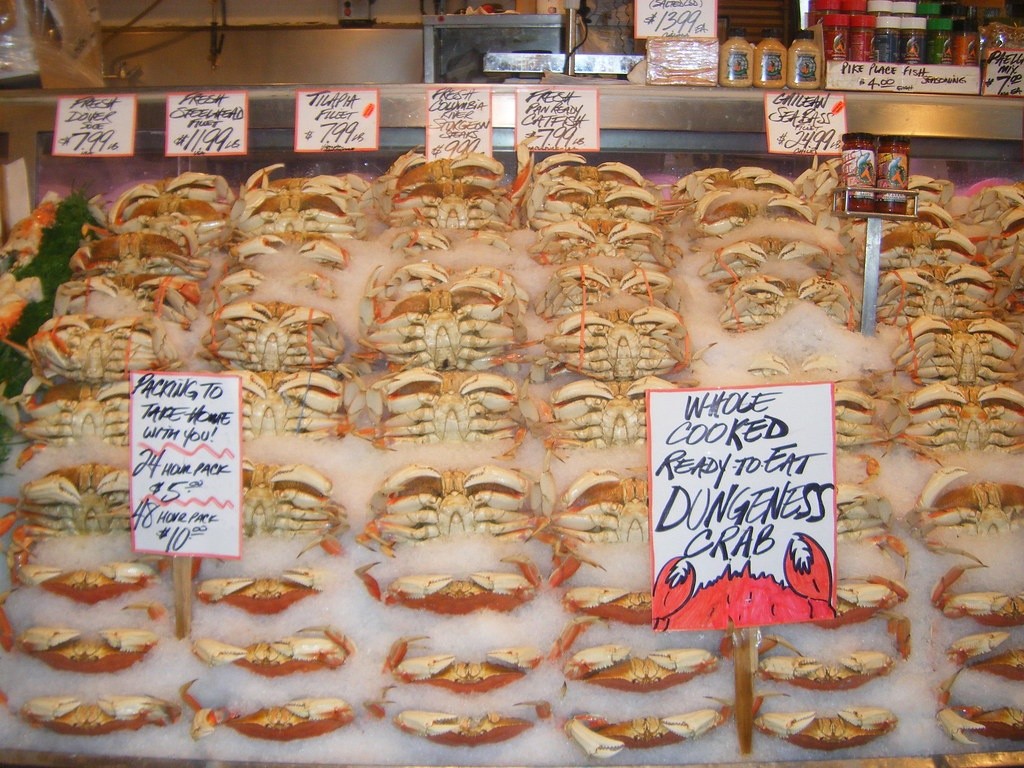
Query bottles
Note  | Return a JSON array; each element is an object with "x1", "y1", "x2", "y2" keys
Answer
[
  {"x1": 787, "y1": 30, "x2": 824, "y2": 89},
  {"x1": 751, "y1": 27, "x2": 788, "y2": 89},
  {"x1": 717, "y1": 27, "x2": 755, "y2": 88},
  {"x1": 806, "y1": 0, "x2": 1001, "y2": 66}
]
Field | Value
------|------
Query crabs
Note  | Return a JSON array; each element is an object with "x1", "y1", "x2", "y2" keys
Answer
[{"x1": 0, "y1": 135, "x2": 1024, "y2": 768}]
